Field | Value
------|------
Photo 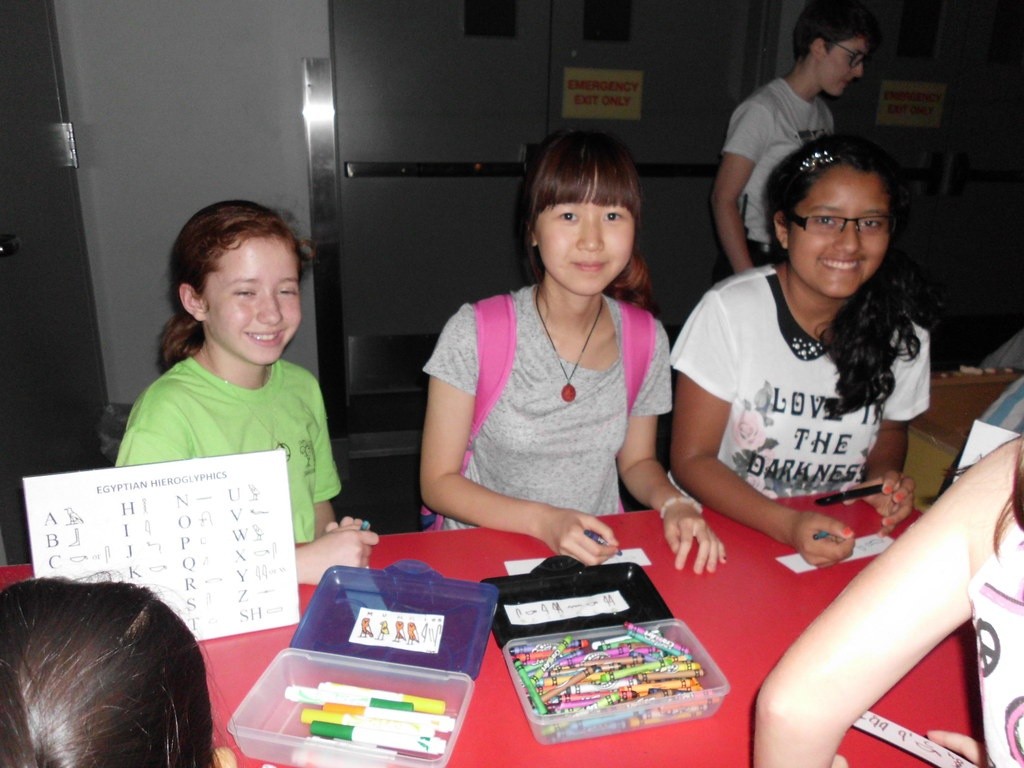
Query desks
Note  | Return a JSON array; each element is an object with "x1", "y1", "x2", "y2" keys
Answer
[
  {"x1": 0, "y1": 489, "x2": 990, "y2": 768},
  {"x1": 901, "y1": 363, "x2": 1024, "y2": 514}
]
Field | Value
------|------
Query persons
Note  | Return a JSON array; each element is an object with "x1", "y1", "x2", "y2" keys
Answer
[
  {"x1": 113, "y1": 199, "x2": 379, "y2": 587},
  {"x1": 669, "y1": 134, "x2": 930, "y2": 569},
  {"x1": 752, "y1": 432, "x2": 1024, "y2": 768},
  {"x1": 420, "y1": 128, "x2": 727, "y2": 574},
  {"x1": 0, "y1": 578, "x2": 237, "y2": 768},
  {"x1": 711, "y1": 0, "x2": 883, "y2": 284}
]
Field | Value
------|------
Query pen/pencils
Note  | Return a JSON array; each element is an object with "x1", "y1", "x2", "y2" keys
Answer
[
  {"x1": 813, "y1": 531, "x2": 828, "y2": 540},
  {"x1": 360, "y1": 520, "x2": 369, "y2": 531},
  {"x1": 816, "y1": 483, "x2": 884, "y2": 506}
]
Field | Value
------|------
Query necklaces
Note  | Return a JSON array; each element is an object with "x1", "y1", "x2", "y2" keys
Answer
[
  {"x1": 535, "y1": 284, "x2": 602, "y2": 402},
  {"x1": 201, "y1": 350, "x2": 285, "y2": 449}
]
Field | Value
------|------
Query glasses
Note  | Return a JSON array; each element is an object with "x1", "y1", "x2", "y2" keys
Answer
[
  {"x1": 792, "y1": 212, "x2": 898, "y2": 235},
  {"x1": 830, "y1": 41, "x2": 866, "y2": 69}
]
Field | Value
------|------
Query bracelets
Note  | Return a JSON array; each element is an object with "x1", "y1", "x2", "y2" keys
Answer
[{"x1": 660, "y1": 497, "x2": 703, "y2": 519}]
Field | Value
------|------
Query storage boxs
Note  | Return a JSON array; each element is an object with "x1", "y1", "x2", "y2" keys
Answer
[
  {"x1": 482, "y1": 553, "x2": 733, "y2": 745},
  {"x1": 224, "y1": 560, "x2": 500, "y2": 768}
]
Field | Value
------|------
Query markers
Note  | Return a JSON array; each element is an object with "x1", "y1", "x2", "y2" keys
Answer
[{"x1": 280, "y1": 680, "x2": 457, "y2": 768}]
